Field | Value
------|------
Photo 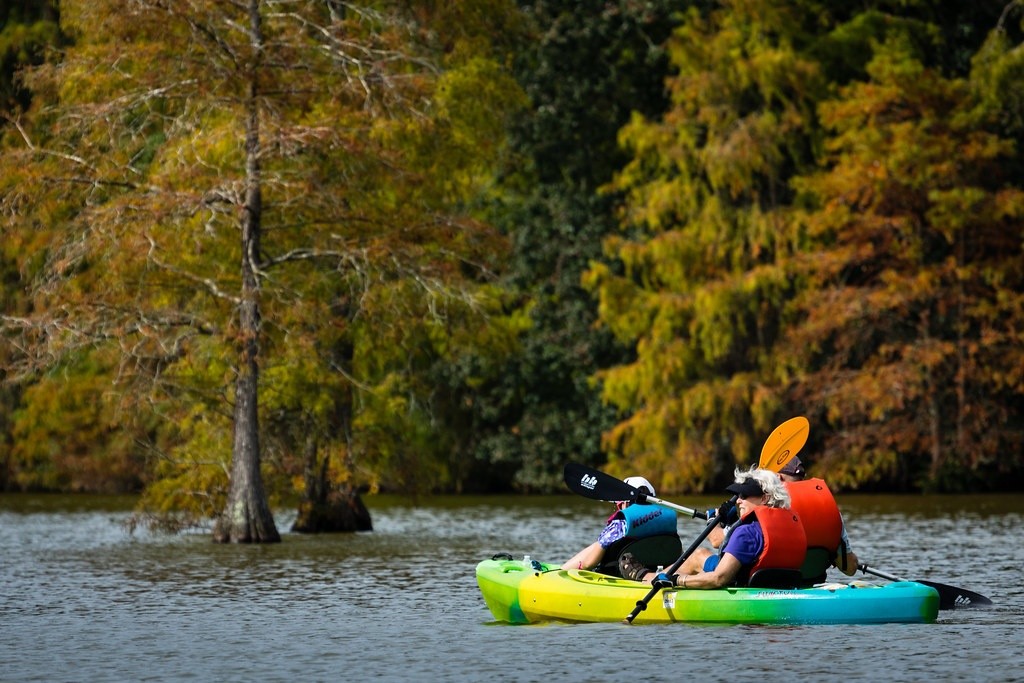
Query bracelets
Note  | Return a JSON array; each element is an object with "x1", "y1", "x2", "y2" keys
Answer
[
  {"x1": 683, "y1": 574, "x2": 689, "y2": 588},
  {"x1": 676, "y1": 574, "x2": 682, "y2": 585}
]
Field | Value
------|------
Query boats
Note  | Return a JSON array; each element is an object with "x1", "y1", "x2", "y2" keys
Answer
[{"x1": 473, "y1": 550, "x2": 942, "y2": 627}]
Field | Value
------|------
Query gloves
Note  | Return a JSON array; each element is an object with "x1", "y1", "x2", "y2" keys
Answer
[
  {"x1": 651, "y1": 574, "x2": 679, "y2": 587},
  {"x1": 707, "y1": 512, "x2": 726, "y2": 529},
  {"x1": 718, "y1": 501, "x2": 739, "y2": 526}
]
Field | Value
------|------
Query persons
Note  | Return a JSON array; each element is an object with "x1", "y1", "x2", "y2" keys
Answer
[
  {"x1": 559, "y1": 477, "x2": 685, "y2": 580},
  {"x1": 651, "y1": 460, "x2": 806, "y2": 592},
  {"x1": 620, "y1": 456, "x2": 859, "y2": 590}
]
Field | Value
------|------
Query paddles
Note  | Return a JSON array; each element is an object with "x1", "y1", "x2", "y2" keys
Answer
[
  {"x1": 855, "y1": 562, "x2": 993, "y2": 609},
  {"x1": 564, "y1": 462, "x2": 714, "y2": 520},
  {"x1": 623, "y1": 416, "x2": 811, "y2": 624}
]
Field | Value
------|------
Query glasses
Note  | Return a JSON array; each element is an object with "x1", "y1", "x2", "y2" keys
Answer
[
  {"x1": 616, "y1": 501, "x2": 624, "y2": 511},
  {"x1": 738, "y1": 492, "x2": 749, "y2": 500}
]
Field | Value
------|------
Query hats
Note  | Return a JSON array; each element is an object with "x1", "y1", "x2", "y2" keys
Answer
[
  {"x1": 777, "y1": 455, "x2": 805, "y2": 477},
  {"x1": 623, "y1": 477, "x2": 656, "y2": 497},
  {"x1": 726, "y1": 477, "x2": 768, "y2": 497}
]
